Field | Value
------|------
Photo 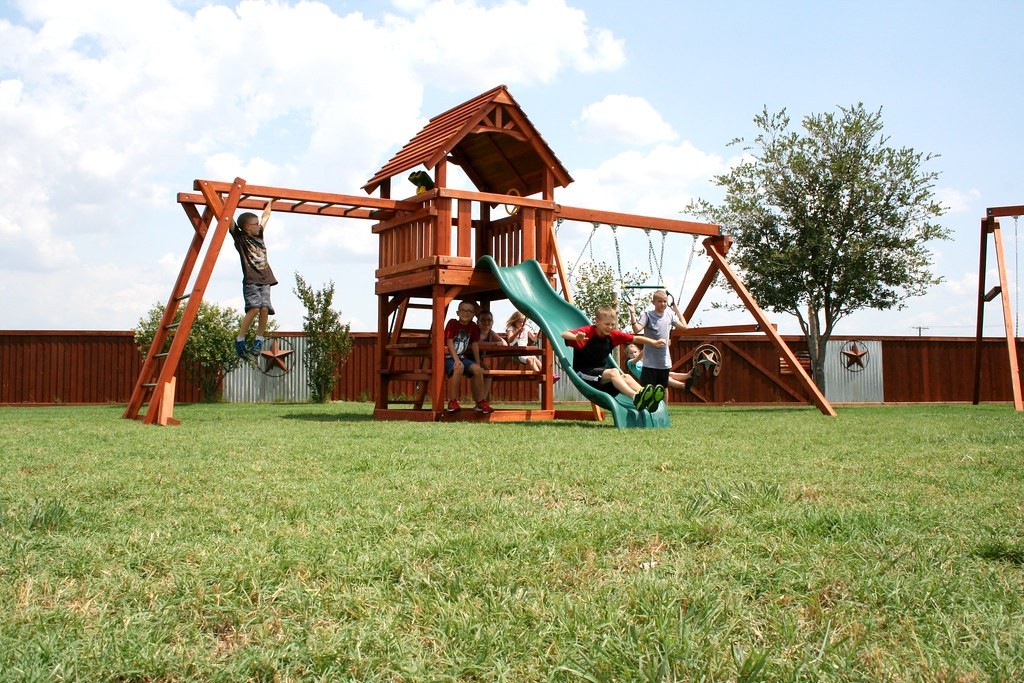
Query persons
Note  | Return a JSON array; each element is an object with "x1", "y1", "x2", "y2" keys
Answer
[
  {"x1": 623, "y1": 289, "x2": 704, "y2": 392},
  {"x1": 217, "y1": 191, "x2": 278, "y2": 361},
  {"x1": 561, "y1": 306, "x2": 671, "y2": 413},
  {"x1": 443, "y1": 300, "x2": 560, "y2": 414}
]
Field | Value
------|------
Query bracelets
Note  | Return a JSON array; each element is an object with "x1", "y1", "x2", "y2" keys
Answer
[
  {"x1": 631, "y1": 322, "x2": 637, "y2": 325},
  {"x1": 675, "y1": 309, "x2": 679, "y2": 315}
]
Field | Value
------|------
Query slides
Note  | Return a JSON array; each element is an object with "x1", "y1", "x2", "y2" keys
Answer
[{"x1": 474, "y1": 255, "x2": 672, "y2": 430}]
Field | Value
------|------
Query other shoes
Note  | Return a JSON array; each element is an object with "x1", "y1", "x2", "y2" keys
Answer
[
  {"x1": 690, "y1": 366, "x2": 699, "y2": 380},
  {"x1": 553, "y1": 374, "x2": 560, "y2": 384},
  {"x1": 685, "y1": 377, "x2": 692, "y2": 393}
]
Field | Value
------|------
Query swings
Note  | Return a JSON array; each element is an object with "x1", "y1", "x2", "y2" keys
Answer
[
  {"x1": 509, "y1": 218, "x2": 598, "y2": 365},
  {"x1": 641, "y1": 231, "x2": 697, "y2": 384},
  {"x1": 611, "y1": 225, "x2": 666, "y2": 289}
]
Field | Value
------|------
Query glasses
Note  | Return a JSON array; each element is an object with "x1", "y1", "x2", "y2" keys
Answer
[
  {"x1": 458, "y1": 308, "x2": 473, "y2": 314},
  {"x1": 250, "y1": 220, "x2": 259, "y2": 225}
]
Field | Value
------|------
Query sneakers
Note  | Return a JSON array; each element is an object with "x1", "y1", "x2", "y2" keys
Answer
[
  {"x1": 474, "y1": 400, "x2": 493, "y2": 413},
  {"x1": 447, "y1": 399, "x2": 461, "y2": 413},
  {"x1": 235, "y1": 338, "x2": 246, "y2": 358},
  {"x1": 633, "y1": 384, "x2": 665, "y2": 413},
  {"x1": 252, "y1": 334, "x2": 263, "y2": 355}
]
